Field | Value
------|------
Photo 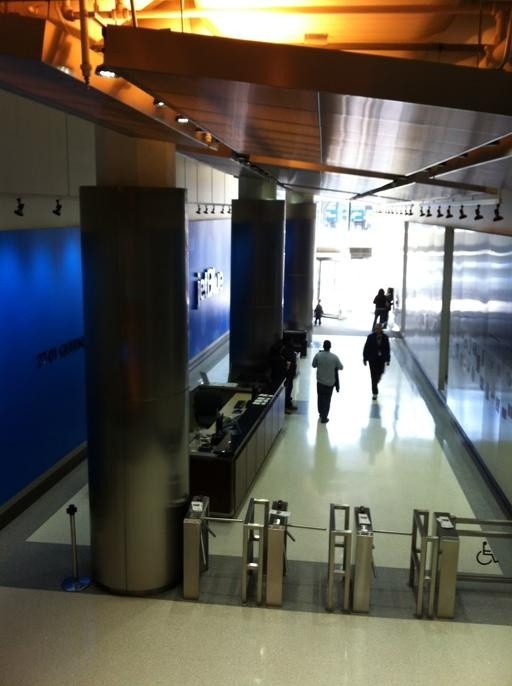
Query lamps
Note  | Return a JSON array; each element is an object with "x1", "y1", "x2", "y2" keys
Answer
[
  {"x1": 3, "y1": 193, "x2": 74, "y2": 220},
  {"x1": 377, "y1": 198, "x2": 504, "y2": 224},
  {"x1": 188, "y1": 203, "x2": 233, "y2": 216}
]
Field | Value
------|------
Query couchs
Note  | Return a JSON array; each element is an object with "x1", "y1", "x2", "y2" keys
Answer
[{"x1": 282, "y1": 329, "x2": 308, "y2": 357}]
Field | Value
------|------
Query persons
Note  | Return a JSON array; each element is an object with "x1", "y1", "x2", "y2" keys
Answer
[
  {"x1": 372, "y1": 289, "x2": 387, "y2": 332},
  {"x1": 381, "y1": 288, "x2": 393, "y2": 329},
  {"x1": 363, "y1": 324, "x2": 390, "y2": 400},
  {"x1": 312, "y1": 340, "x2": 343, "y2": 423},
  {"x1": 279, "y1": 336, "x2": 297, "y2": 410},
  {"x1": 312, "y1": 299, "x2": 324, "y2": 327},
  {"x1": 271, "y1": 341, "x2": 291, "y2": 414}
]
